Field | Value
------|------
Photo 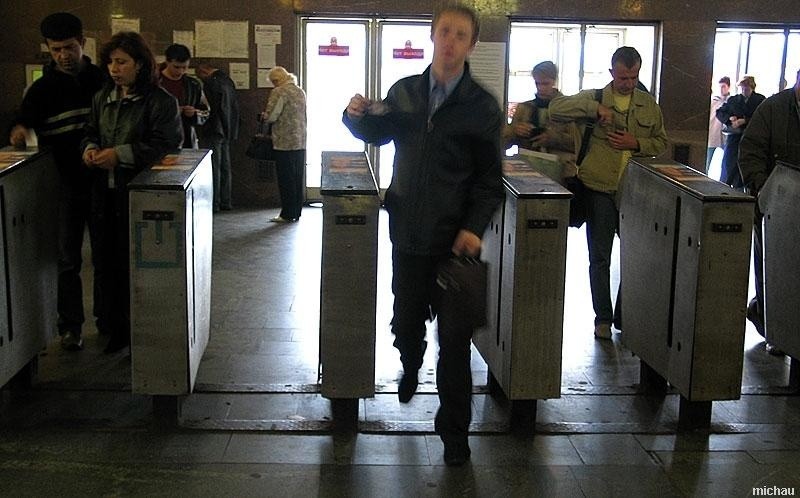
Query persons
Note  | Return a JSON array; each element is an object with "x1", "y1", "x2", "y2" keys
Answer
[
  {"x1": 343, "y1": 5, "x2": 503, "y2": 468},
  {"x1": 196, "y1": 62, "x2": 239, "y2": 211},
  {"x1": 10, "y1": 11, "x2": 111, "y2": 350},
  {"x1": 740, "y1": 70, "x2": 800, "y2": 356},
  {"x1": 502, "y1": 61, "x2": 581, "y2": 173},
  {"x1": 704, "y1": 75, "x2": 732, "y2": 175},
  {"x1": 257, "y1": 67, "x2": 306, "y2": 223},
  {"x1": 716, "y1": 77, "x2": 766, "y2": 188},
  {"x1": 81, "y1": 29, "x2": 182, "y2": 354},
  {"x1": 550, "y1": 46, "x2": 667, "y2": 339},
  {"x1": 157, "y1": 44, "x2": 211, "y2": 150}
]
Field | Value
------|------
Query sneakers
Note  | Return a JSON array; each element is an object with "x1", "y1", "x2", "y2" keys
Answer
[
  {"x1": 593, "y1": 322, "x2": 613, "y2": 342},
  {"x1": 762, "y1": 337, "x2": 788, "y2": 358},
  {"x1": 746, "y1": 296, "x2": 765, "y2": 337},
  {"x1": 101, "y1": 328, "x2": 131, "y2": 357},
  {"x1": 267, "y1": 215, "x2": 292, "y2": 224},
  {"x1": 439, "y1": 430, "x2": 473, "y2": 469},
  {"x1": 397, "y1": 363, "x2": 419, "y2": 405},
  {"x1": 59, "y1": 328, "x2": 85, "y2": 351}
]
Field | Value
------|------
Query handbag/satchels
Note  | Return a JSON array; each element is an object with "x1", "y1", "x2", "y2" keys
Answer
[
  {"x1": 430, "y1": 249, "x2": 492, "y2": 343},
  {"x1": 565, "y1": 174, "x2": 593, "y2": 230},
  {"x1": 722, "y1": 119, "x2": 746, "y2": 137},
  {"x1": 245, "y1": 129, "x2": 278, "y2": 162}
]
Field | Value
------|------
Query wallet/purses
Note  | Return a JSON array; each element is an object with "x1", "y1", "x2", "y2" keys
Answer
[{"x1": 526, "y1": 124, "x2": 545, "y2": 139}]
